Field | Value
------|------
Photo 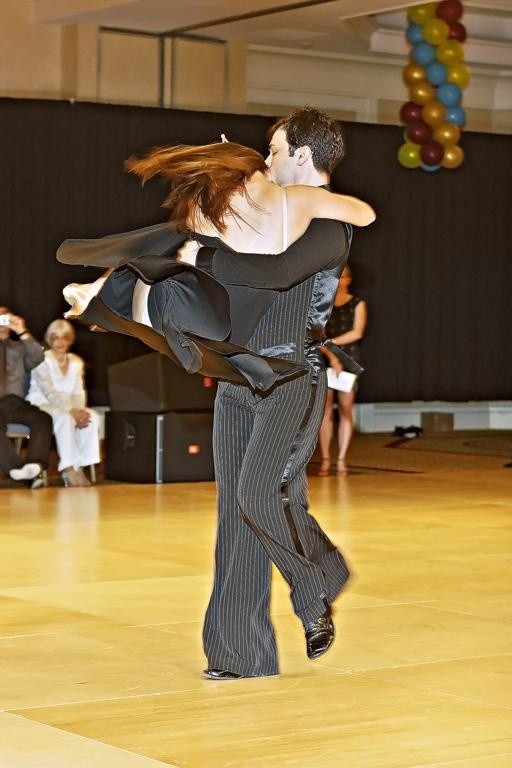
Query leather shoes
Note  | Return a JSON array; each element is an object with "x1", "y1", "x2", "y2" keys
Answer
[
  {"x1": 304, "y1": 601, "x2": 336, "y2": 660},
  {"x1": 202, "y1": 667, "x2": 246, "y2": 681}
]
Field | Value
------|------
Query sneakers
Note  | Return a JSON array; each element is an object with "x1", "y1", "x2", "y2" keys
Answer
[{"x1": 9, "y1": 463, "x2": 44, "y2": 490}]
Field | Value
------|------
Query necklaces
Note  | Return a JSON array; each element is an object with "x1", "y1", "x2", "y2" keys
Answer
[{"x1": 57, "y1": 352, "x2": 69, "y2": 368}]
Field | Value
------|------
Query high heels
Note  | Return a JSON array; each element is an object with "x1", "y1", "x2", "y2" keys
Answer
[
  {"x1": 317, "y1": 457, "x2": 350, "y2": 477},
  {"x1": 60, "y1": 276, "x2": 99, "y2": 321},
  {"x1": 61, "y1": 471, "x2": 92, "y2": 488}
]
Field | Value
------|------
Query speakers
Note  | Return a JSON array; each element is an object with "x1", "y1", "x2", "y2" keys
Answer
[
  {"x1": 107, "y1": 352, "x2": 219, "y2": 412},
  {"x1": 105, "y1": 412, "x2": 216, "y2": 484}
]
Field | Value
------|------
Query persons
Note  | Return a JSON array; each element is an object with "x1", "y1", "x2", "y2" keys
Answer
[
  {"x1": 56, "y1": 132, "x2": 382, "y2": 406},
  {"x1": 313, "y1": 262, "x2": 368, "y2": 480},
  {"x1": 175, "y1": 103, "x2": 353, "y2": 685},
  {"x1": 24, "y1": 317, "x2": 103, "y2": 489},
  {"x1": 0, "y1": 301, "x2": 55, "y2": 489}
]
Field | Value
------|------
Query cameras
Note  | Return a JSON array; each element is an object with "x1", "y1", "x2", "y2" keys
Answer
[{"x1": 0, "y1": 315, "x2": 10, "y2": 325}]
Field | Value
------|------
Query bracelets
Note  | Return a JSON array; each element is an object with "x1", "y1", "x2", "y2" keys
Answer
[
  {"x1": 17, "y1": 329, "x2": 29, "y2": 339},
  {"x1": 331, "y1": 360, "x2": 340, "y2": 368}
]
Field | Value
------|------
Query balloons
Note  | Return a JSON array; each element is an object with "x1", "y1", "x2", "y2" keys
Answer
[{"x1": 393, "y1": 2, "x2": 470, "y2": 179}]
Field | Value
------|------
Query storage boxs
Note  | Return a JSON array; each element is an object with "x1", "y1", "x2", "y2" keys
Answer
[{"x1": 422, "y1": 412, "x2": 453, "y2": 433}]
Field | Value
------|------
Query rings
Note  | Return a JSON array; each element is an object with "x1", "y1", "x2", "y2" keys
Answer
[{"x1": 80, "y1": 418, "x2": 83, "y2": 422}]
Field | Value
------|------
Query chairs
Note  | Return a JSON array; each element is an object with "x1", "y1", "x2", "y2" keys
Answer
[{"x1": 6, "y1": 375, "x2": 96, "y2": 488}]
